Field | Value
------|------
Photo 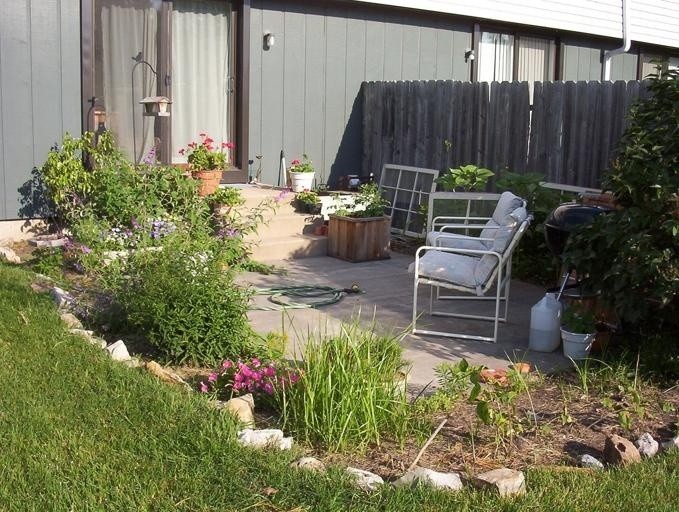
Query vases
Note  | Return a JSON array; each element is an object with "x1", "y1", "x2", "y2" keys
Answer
[
  {"x1": 191, "y1": 170, "x2": 223, "y2": 198},
  {"x1": 299, "y1": 202, "x2": 308, "y2": 214}
]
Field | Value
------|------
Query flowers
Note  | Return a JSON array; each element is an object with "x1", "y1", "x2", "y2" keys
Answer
[
  {"x1": 178, "y1": 133, "x2": 236, "y2": 178},
  {"x1": 297, "y1": 188, "x2": 311, "y2": 202},
  {"x1": 289, "y1": 154, "x2": 315, "y2": 173}
]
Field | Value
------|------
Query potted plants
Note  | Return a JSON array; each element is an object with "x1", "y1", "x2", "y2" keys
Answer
[
  {"x1": 560, "y1": 303, "x2": 600, "y2": 359},
  {"x1": 328, "y1": 178, "x2": 394, "y2": 263},
  {"x1": 308, "y1": 192, "x2": 323, "y2": 214},
  {"x1": 209, "y1": 186, "x2": 246, "y2": 218}
]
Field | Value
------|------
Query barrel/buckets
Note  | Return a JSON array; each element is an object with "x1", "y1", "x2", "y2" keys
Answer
[
  {"x1": 559, "y1": 327, "x2": 599, "y2": 360},
  {"x1": 528, "y1": 292, "x2": 562, "y2": 352}
]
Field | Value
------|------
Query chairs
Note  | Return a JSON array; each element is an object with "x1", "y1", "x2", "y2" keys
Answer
[{"x1": 408, "y1": 191, "x2": 534, "y2": 343}]
